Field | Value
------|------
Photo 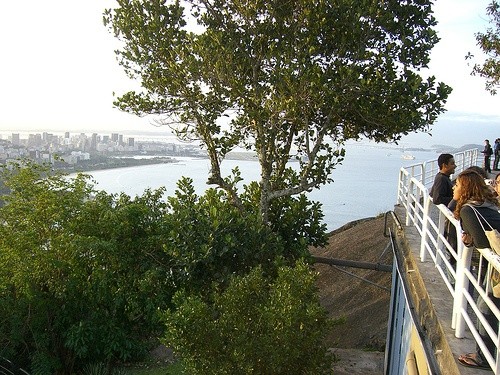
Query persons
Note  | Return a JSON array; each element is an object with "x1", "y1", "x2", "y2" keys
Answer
[
  {"x1": 451, "y1": 169, "x2": 499, "y2": 370},
  {"x1": 479, "y1": 138, "x2": 492, "y2": 174},
  {"x1": 493, "y1": 136, "x2": 500, "y2": 171},
  {"x1": 428, "y1": 152, "x2": 500, "y2": 286}
]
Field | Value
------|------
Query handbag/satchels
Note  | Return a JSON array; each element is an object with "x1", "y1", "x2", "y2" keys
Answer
[
  {"x1": 484, "y1": 229, "x2": 500, "y2": 299},
  {"x1": 487, "y1": 148, "x2": 493, "y2": 154}
]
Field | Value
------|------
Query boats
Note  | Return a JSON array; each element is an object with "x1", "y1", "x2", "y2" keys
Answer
[{"x1": 400, "y1": 152, "x2": 416, "y2": 160}]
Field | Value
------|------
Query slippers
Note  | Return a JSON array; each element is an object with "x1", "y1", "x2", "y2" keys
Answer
[{"x1": 458, "y1": 354, "x2": 492, "y2": 370}]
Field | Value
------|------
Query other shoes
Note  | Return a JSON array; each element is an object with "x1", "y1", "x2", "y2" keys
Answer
[{"x1": 494, "y1": 169, "x2": 499, "y2": 171}]
